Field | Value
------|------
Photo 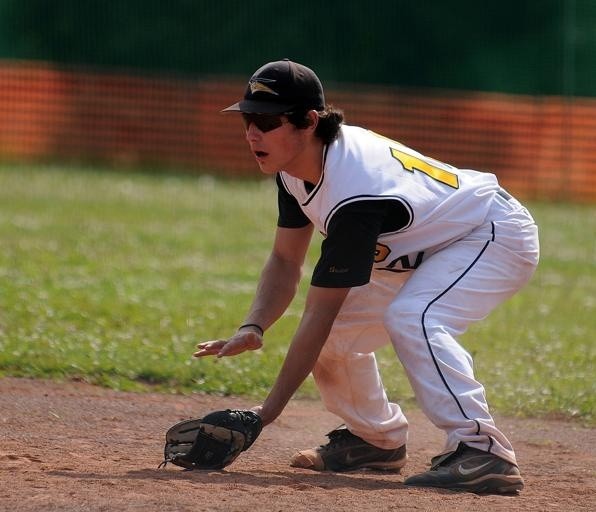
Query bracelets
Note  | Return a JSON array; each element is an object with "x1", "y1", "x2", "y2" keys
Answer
[{"x1": 238, "y1": 319, "x2": 266, "y2": 339}]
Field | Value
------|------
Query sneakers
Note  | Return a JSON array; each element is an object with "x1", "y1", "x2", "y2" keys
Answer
[
  {"x1": 292, "y1": 424, "x2": 408, "y2": 471},
  {"x1": 403, "y1": 441, "x2": 524, "y2": 492}
]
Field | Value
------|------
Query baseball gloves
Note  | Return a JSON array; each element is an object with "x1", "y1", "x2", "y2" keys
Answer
[{"x1": 159, "y1": 409, "x2": 262, "y2": 470}]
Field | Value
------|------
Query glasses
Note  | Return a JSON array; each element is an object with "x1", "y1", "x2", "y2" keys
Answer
[{"x1": 242, "y1": 113, "x2": 296, "y2": 133}]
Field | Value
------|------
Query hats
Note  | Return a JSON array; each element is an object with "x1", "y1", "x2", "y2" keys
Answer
[{"x1": 222, "y1": 58, "x2": 325, "y2": 113}]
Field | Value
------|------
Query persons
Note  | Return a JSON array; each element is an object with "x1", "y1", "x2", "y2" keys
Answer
[{"x1": 185, "y1": 58, "x2": 544, "y2": 495}]
